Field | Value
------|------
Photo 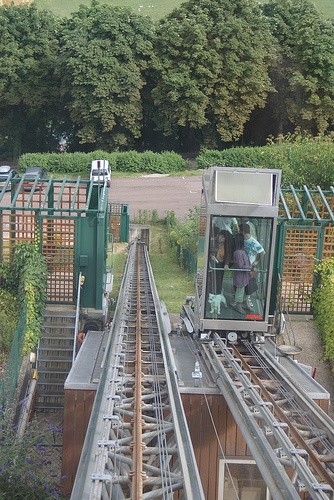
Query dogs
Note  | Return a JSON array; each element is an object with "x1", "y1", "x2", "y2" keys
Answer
[{"x1": 208, "y1": 288, "x2": 228, "y2": 314}]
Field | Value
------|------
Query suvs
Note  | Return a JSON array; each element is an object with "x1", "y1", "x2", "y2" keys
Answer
[{"x1": 90, "y1": 160, "x2": 112, "y2": 188}]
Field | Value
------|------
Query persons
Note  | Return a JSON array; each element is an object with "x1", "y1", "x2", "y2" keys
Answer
[
  {"x1": 207, "y1": 230, "x2": 233, "y2": 295},
  {"x1": 230, "y1": 223, "x2": 266, "y2": 308},
  {"x1": 213, "y1": 226, "x2": 220, "y2": 240},
  {"x1": 240, "y1": 217, "x2": 257, "y2": 240},
  {"x1": 233, "y1": 234, "x2": 254, "y2": 315},
  {"x1": 212, "y1": 215, "x2": 240, "y2": 236}
]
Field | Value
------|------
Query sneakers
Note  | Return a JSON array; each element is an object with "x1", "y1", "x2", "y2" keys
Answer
[
  {"x1": 230, "y1": 296, "x2": 237, "y2": 306},
  {"x1": 235, "y1": 305, "x2": 245, "y2": 314},
  {"x1": 247, "y1": 299, "x2": 254, "y2": 307},
  {"x1": 245, "y1": 306, "x2": 254, "y2": 311}
]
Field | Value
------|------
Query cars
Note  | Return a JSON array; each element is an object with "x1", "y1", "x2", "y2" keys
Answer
[
  {"x1": 0, "y1": 165, "x2": 21, "y2": 191},
  {"x1": 22, "y1": 167, "x2": 48, "y2": 191}
]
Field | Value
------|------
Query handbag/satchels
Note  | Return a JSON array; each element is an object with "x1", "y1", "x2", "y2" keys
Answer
[{"x1": 245, "y1": 277, "x2": 258, "y2": 295}]
